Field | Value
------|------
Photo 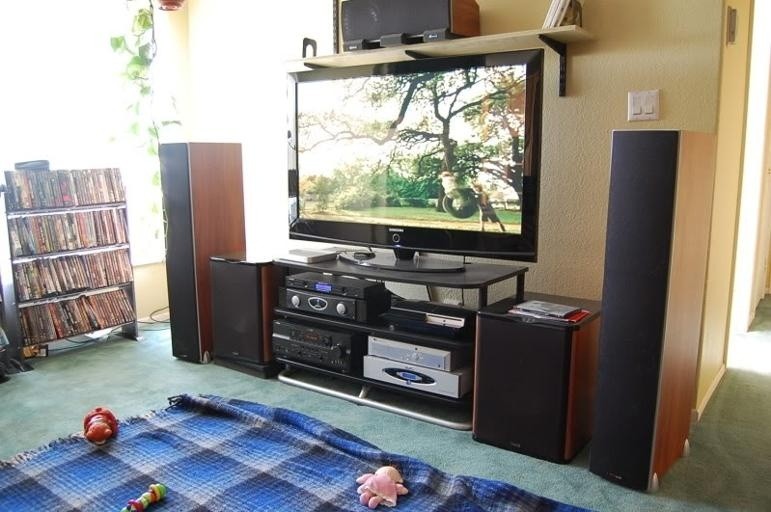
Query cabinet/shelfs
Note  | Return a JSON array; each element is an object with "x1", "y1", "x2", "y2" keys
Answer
[
  {"x1": 273, "y1": 247, "x2": 530, "y2": 432},
  {"x1": 1, "y1": 168, "x2": 138, "y2": 364}
]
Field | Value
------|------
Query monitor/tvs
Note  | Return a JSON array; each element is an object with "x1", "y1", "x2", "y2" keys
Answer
[{"x1": 284, "y1": 47, "x2": 545, "y2": 273}]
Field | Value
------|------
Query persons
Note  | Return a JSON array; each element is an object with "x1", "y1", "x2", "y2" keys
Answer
[
  {"x1": 441, "y1": 171, "x2": 463, "y2": 211},
  {"x1": 471, "y1": 183, "x2": 506, "y2": 233}
]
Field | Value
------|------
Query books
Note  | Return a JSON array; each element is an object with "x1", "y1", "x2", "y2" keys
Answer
[
  {"x1": 541, "y1": 0, "x2": 586, "y2": 29},
  {"x1": 508, "y1": 307, "x2": 592, "y2": 322},
  {"x1": 2, "y1": 166, "x2": 137, "y2": 347},
  {"x1": 513, "y1": 299, "x2": 583, "y2": 318}
]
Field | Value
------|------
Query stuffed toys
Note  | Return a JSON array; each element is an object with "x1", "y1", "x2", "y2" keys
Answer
[
  {"x1": 120, "y1": 484, "x2": 166, "y2": 512},
  {"x1": 355, "y1": 466, "x2": 409, "y2": 510}
]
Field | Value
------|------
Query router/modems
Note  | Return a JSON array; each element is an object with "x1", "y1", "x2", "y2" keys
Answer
[{"x1": 278, "y1": 285, "x2": 392, "y2": 322}]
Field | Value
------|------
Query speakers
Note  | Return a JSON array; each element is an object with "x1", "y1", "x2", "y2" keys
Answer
[
  {"x1": 337, "y1": 0, "x2": 481, "y2": 52},
  {"x1": 470, "y1": 291, "x2": 601, "y2": 466},
  {"x1": 208, "y1": 245, "x2": 274, "y2": 366},
  {"x1": 156, "y1": 140, "x2": 247, "y2": 365},
  {"x1": 590, "y1": 127, "x2": 716, "y2": 494}
]
